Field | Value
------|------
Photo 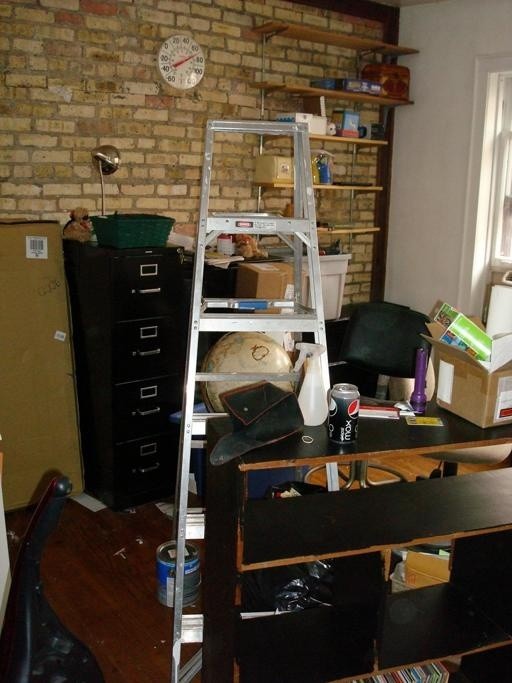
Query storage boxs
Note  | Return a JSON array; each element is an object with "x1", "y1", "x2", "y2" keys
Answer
[
  {"x1": 418, "y1": 325, "x2": 511, "y2": 427},
  {"x1": 235, "y1": 260, "x2": 303, "y2": 298}
]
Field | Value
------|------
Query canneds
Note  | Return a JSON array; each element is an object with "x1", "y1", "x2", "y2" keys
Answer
[{"x1": 325, "y1": 383, "x2": 360, "y2": 444}]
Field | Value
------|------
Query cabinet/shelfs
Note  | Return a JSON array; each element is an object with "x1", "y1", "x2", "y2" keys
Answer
[
  {"x1": 256, "y1": 36, "x2": 421, "y2": 283},
  {"x1": 203, "y1": 404, "x2": 512, "y2": 683},
  {"x1": 64, "y1": 237, "x2": 188, "y2": 507}
]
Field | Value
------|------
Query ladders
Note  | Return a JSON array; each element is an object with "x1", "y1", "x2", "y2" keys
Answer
[{"x1": 172, "y1": 120, "x2": 340, "y2": 680}]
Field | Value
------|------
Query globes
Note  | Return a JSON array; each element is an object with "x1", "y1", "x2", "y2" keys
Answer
[{"x1": 203, "y1": 332, "x2": 295, "y2": 412}]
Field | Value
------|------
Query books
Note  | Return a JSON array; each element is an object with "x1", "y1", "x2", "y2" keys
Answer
[
  {"x1": 359, "y1": 396, "x2": 400, "y2": 411},
  {"x1": 359, "y1": 410, "x2": 399, "y2": 420},
  {"x1": 352, "y1": 661, "x2": 449, "y2": 683}
]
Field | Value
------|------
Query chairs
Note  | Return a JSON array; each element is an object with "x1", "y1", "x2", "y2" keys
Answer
[{"x1": 353, "y1": 308, "x2": 433, "y2": 376}]
